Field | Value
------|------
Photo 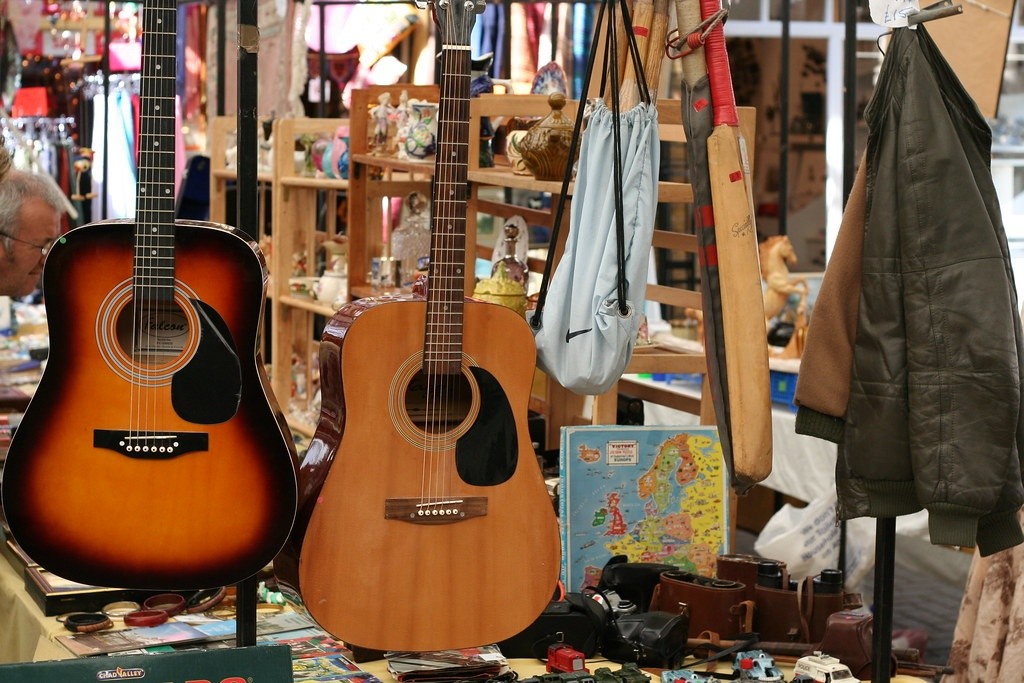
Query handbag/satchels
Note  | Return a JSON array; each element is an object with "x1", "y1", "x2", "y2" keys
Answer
[{"x1": 600, "y1": 554, "x2": 898, "y2": 680}]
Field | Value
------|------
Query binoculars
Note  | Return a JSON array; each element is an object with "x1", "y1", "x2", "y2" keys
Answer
[
  {"x1": 756, "y1": 559, "x2": 843, "y2": 595},
  {"x1": 665, "y1": 569, "x2": 738, "y2": 589}
]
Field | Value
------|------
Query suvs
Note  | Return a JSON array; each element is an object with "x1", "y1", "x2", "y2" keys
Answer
[{"x1": 545, "y1": 644, "x2": 865, "y2": 683}]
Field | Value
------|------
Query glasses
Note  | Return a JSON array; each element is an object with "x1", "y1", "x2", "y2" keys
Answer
[{"x1": 0, "y1": 230, "x2": 52, "y2": 255}]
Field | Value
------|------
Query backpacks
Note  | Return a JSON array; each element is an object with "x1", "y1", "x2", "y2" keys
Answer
[{"x1": 535, "y1": 98, "x2": 658, "y2": 393}]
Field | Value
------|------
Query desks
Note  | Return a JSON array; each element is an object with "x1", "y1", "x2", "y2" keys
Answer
[{"x1": 0, "y1": 368, "x2": 931, "y2": 683}]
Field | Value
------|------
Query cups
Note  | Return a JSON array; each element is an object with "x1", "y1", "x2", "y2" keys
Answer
[{"x1": 316, "y1": 264, "x2": 346, "y2": 304}]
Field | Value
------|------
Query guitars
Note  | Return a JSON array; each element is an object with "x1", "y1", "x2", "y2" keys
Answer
[
  {"x1": 1, "y1": 0, "x2": 303, "y2": 593},
  {"x1": 268, "y1": 1, "x2": 564, "y2": 655}
]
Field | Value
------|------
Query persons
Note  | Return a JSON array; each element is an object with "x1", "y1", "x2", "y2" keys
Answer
[
  {"x1": 0, "y1": 171, "x2": 67, "y2": 296},
  {"x1": 368, "y1": 93, "x2": 408, "y2": 146}
]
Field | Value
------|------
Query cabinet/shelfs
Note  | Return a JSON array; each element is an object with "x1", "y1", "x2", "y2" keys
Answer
[
  {"x1": 208, "y1": 114, "x2": 347, "y2": 410},
  {"x1": 346, "y1": 86, "x2": 571, "y2": 305},
  {"x1": 277, "y1": 118, "x2": 431, "y2": 437},
  {"x1": 469, "y1": 98, "x2": 757, "y2": 451}
]
